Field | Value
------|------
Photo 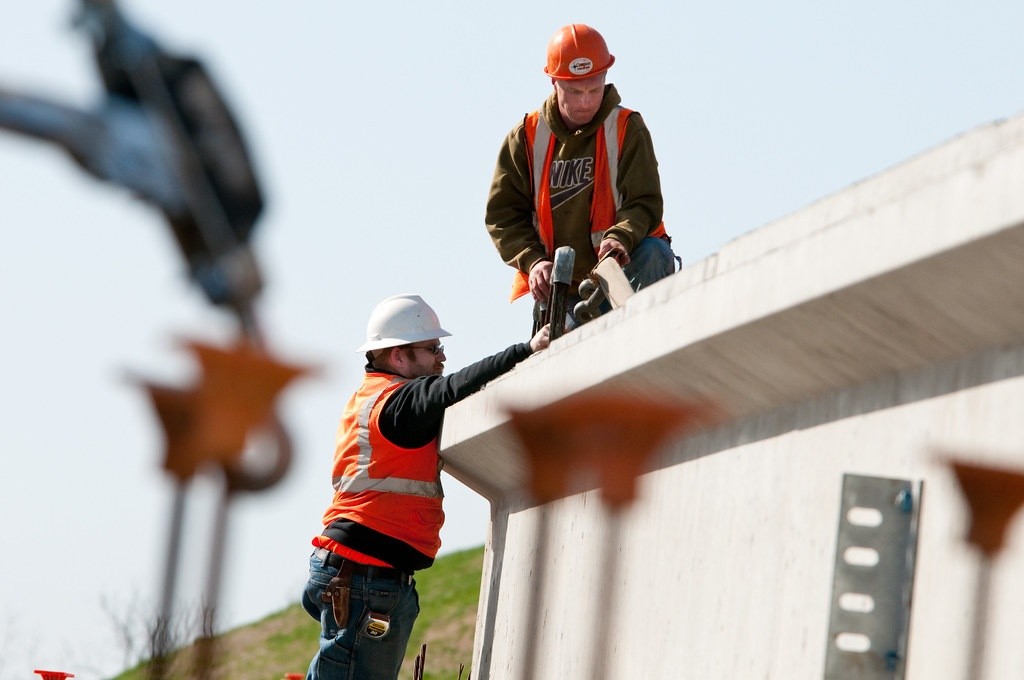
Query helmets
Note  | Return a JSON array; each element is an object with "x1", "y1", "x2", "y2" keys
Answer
[
  {"x1": 357, "y1": 294, "x2": 452, "y2": 352},
  {"x1": 544, "y1": 24, "x2": 615, "y2": 80}
]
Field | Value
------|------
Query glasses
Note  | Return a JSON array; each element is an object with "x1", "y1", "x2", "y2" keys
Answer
[{"x1": 398, "y1": 346, "x2": 444, "y2": 356}]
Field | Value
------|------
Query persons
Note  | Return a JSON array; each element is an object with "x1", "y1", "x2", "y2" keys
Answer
[
  {"x1": 302, "y1": 295, "x2": 571, "y2": 680},
  {"x1": 485, "y1": 24, "x2": 677, "y2": 333}
]
